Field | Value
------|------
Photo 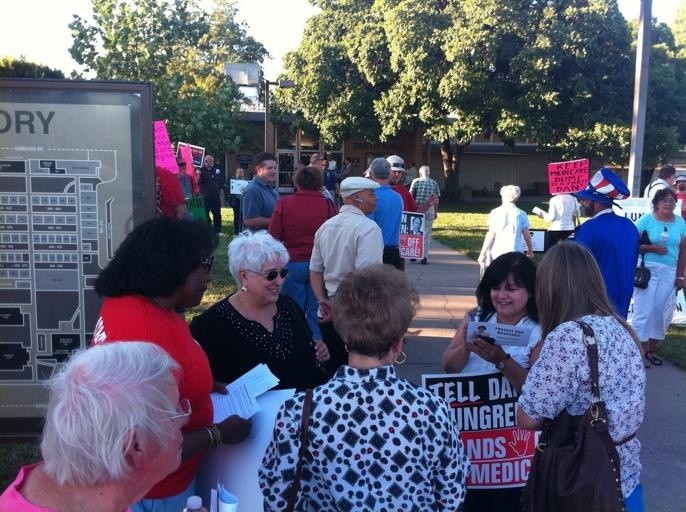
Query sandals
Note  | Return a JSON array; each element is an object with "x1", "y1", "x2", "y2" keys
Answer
[{"x1": 644, "y1": 351, "x2": 662, "y2": 365}]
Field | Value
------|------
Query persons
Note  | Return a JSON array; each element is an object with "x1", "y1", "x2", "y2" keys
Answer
[
  {"x1": 514, "y1": 240, "x2": 647, "y2": 511},
  {"x1": 1, "y1": 342, "x2": 191, "y2": 511},
  {"x1": 191, "y1": 229, "x2": 330, "y2": 397},
  {"x1": 88, "y1": 220, "x2": 253, "y2": 510},
  {"x1": 156, "y1": 154, "x2": 441, "y2": 377},
  {"x1": 257, "y1": 262, "x2": 470, "y2": 512},
  {"x1": 442, "y1": 164, "x2": 686, "y2": 375}
]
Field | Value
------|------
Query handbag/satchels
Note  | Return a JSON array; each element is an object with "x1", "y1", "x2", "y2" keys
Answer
[
  {"x1": 522, "y1": 402, "x2": 626, "y2": 512},
  {"x1": 634, "y1": 266, "x2": 651, "y2": 288}
]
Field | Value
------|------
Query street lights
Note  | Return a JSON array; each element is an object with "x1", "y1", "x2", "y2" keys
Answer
[{"x1": 263, "y1": 79, "x2": 297, "y2": 156}]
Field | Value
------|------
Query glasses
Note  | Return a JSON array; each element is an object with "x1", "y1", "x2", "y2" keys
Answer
[
  {"x1": 245, "y1": 267, "x2": 289, "y2": 281},
  {"x1": 200, "y1": 255, "x2": 214, "y2": 272},
  {"x1": 392, "y1": 163, "x2": 404, "y2": 168}
]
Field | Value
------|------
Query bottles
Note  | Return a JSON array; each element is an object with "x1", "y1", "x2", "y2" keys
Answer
[
  {"x1": 179, "y1": 495, "x2": 211, "y2": 512},
  {"x1": 658, "y1": 226, "x2": 671, "y2": 253},
  {"x1": 323, "y1": 186, "x2": 333, "y2": 200}
]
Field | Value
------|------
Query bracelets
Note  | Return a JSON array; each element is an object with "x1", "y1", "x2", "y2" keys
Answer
[{"x1": 207, "y1": 425, "x2": 221, "y2": 448}]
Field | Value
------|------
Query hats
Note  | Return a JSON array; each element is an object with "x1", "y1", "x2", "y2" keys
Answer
[
  {"x1": 387, "y1": 155, "x2": 405, "y2": 172},
  {"x1": 573, "y1": 168, "x2": 630, "y2": 206},
  {"x1": 339, "y1": 176, "x2": 380, "y2": 197},
  {"x1": 369, "y1": 158, "x2": 390, "y2": 180}
]
